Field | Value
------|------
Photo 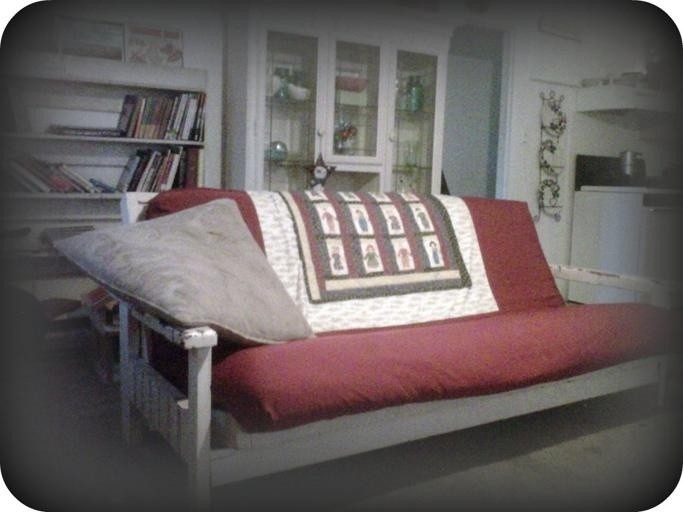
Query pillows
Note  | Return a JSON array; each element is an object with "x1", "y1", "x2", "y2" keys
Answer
[{"x1": 52, "y1": 194, "x2": 317, "y2": 348}]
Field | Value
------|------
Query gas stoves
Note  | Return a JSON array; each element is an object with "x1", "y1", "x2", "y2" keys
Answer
[{"x1": 581, "y1": 183, "x2": 682, "y2": 195}]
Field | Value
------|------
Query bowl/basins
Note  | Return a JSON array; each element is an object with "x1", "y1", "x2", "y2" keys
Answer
[
  {"x1": 287, "y1": 85, "x2": 310, "y2": 101},
  {"x1": 337, "y1": 76, "x2": 369, "y2": 92}
]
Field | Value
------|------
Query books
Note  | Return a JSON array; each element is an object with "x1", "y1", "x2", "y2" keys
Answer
[
  {"x1": 49, "y1": 92, "x2": 207, "y2": 141},
  {"x1": 1, "y1": 147, "x2": 199, "y2": 193}
]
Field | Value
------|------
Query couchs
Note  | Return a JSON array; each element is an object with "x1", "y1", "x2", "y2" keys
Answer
[{"x1": 114, "y1": 185, "x2": 680, "y2": 509}]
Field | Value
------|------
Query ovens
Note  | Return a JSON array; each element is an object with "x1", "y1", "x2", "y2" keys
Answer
[{"x1": 640, "y1": 205, "x2": 683, "y2": 312}]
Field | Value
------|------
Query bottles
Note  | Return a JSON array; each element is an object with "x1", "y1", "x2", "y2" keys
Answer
[{"x1": 406, "y1": 77, "x2": 424, "y2": 112}]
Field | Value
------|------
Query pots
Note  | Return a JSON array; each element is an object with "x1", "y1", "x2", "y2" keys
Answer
[{"x1": 616, "y1": 150, "x2": 642, "y2": 178}]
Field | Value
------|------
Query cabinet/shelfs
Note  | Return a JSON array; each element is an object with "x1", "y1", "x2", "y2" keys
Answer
[
  {"x1": 0, "y1": 49, "x2": 214, "y2": 344},
  {"x1": 223, "y1": 2, "x2": 467, "y2": 197}
]
